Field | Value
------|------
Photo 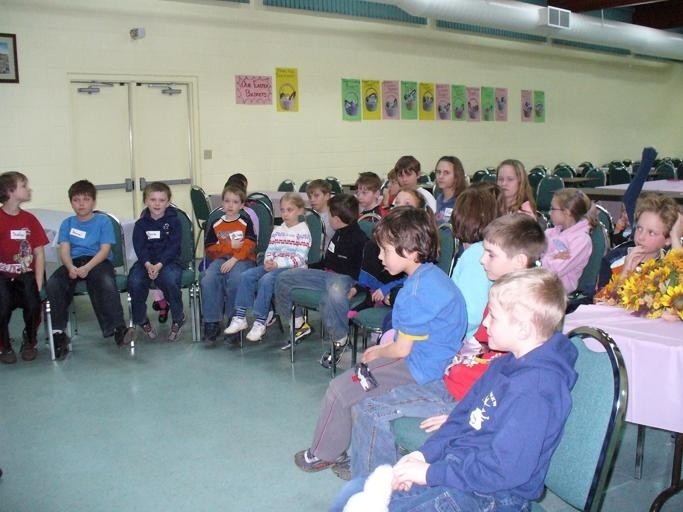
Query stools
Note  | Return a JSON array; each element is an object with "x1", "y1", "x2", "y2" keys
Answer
[{"x1": 389, "y1": 416, "x2": 440, "y2": 456}]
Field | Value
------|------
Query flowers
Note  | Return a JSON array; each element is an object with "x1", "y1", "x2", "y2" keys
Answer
[{"x1": 602, "y1": 239, "x2": 683, "y2": 323}]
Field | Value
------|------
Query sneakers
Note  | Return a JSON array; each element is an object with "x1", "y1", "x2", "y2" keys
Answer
[
  {"x1": 320, "y1": 337, "x2": 349, "y2": 368},
  {"x1": 204, "y1": 316, "x2": 313, "y2": 349},
  {"x1": 1, "y1": 329, "x2": 70, "y2": 363},
  {"x1": 115, "y1": 326, "x2": 135, "y2": 347},
  {"x1": 294, "y1": 448, "x2": 353, "y2": 481},
  {"x1": 168, "y1": 324, "x2": 183, "y2": 342},
  {"x1": 143, "y1": 323, "x2": 158, "y2": 340}
]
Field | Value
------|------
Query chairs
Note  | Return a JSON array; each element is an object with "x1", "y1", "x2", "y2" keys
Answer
[
  {"x1": 0, "y1": 207, "x2": 194, "y2": 355},
  {"x1": 192, "y1": 154, "x2": 604, "y2": 373},
  {"x1": 535, "y1": 326, "x2": 629, "y2": 512},
  {"x1": 606, "y1": 154, "x2": 681, "y2": 255}
]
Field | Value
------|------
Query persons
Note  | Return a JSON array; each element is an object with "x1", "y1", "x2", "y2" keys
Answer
[
  {"x1": 349, "y1": 213, "x2": 548, "y2": 480},
  {"x1": 195, "y1": 152, "x2": 598, "y2": 368},
  {"x1": 45, "y1": 180, "x2": 137, "y2": 360},
  {"x1": 0, "y1": 171, "x2": 50, "y2": 365},
  {"x1": 151, "y1": 286, "x2": 172, "y2": 323},
  {"x1": 126, "y1": 180, "x2": 195, "y2": 343},
  {"x1": 292, "y1": 204, "x2": 469, "y2": 482},
  {"x1": 595, "y1": 191, "x2": 682, "y2": 293},
  {"x1": 340, "y1": 265, "x2": 581, "y2": 512}
]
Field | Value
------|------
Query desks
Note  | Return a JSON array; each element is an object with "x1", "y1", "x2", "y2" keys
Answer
[{"x1": 549, "y1": 285, "x2": 681, "y2": 511}]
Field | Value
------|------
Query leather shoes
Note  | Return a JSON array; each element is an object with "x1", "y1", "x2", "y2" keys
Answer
[
  {"x1": 153, "y1": 301, "x2": 160, "y2": 311},
  {"x1": 159, "y1": 304, "x2": 169, "y2": 323}
]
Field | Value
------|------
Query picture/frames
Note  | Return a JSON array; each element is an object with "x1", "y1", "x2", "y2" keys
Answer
[{"x1": 0, "y1": 29, "x2": 20, "y2": 84}]
